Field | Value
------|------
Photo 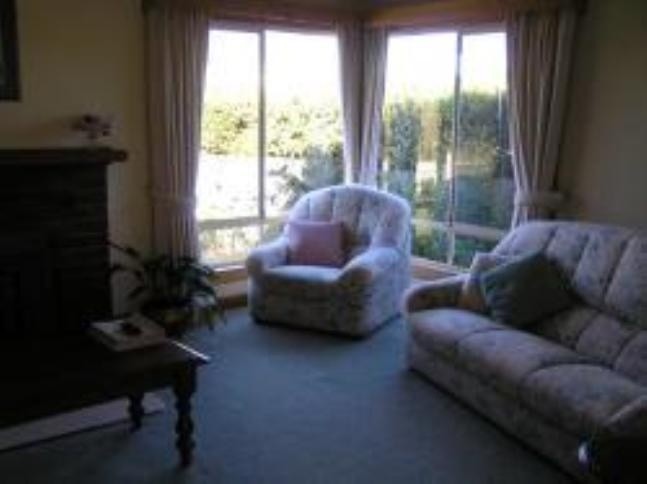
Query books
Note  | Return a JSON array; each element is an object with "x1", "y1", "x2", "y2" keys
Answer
[
  {"x1": 89, "y1": 311, "x2": 165, "y2": 342},
  {"x1": 87, "y1": 327, "x2": 165, "y2": 352}
]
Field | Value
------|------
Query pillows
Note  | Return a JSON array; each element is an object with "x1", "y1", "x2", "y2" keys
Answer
[
  {"x1": 475, "y1": 242, "x2": 566, "y2": 330},
  {"x1": 456, "y1": 247, "x2": 513, "y2": 314},
  {"x1": 281, "y1": 215, "x2": 348, "y2": 269}
]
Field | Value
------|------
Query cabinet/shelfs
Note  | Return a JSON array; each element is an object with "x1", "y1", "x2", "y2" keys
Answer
[{"x1": 0, "y1": 143, "x2": 131, "y2": 323}]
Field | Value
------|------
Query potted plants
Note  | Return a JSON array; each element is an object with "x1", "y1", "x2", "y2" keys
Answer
[{"x1": 105, "y1": 229, "x2": 227, "y2": 337}]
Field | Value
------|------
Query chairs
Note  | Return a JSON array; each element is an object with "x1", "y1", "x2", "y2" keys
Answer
[{"x1": 242, "y1": 180, "x2": 413, "y2": 342}]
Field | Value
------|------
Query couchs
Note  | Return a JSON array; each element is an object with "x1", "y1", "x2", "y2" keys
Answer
[{"x1": 393, "y1": 214, "x2": 646, "y2": 483}]
